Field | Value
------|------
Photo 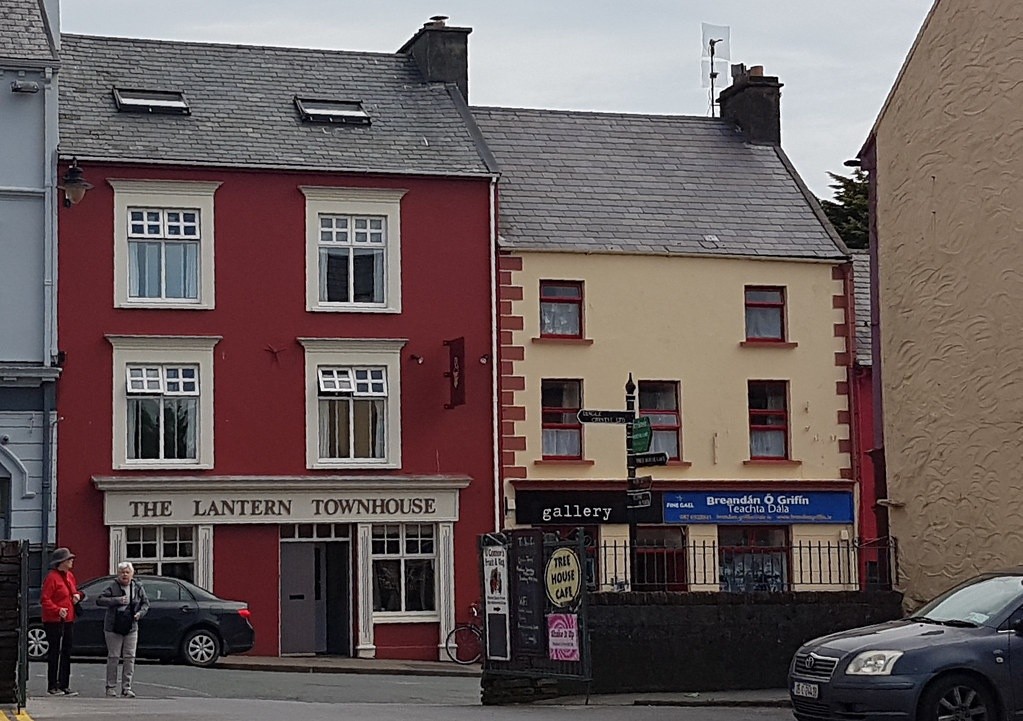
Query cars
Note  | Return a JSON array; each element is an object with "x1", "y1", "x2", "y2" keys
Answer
[
  {"x1": 787, "y1": 572, "x2": 1023, "y2": 721},
  {"x1": 25, "y1": 572, "x2": 258, "y2": 667}
]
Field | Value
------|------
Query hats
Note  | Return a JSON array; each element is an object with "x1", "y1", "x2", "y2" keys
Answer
[{"x1": 49, "y1": 548, "x2": 76, "y2": 564}]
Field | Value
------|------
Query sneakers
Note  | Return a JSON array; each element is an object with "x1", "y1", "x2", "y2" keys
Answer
[
  {"x1": 60, "y1": 688, "x2": 78, "y2": 697},
  {"x1": 106, "y1": 686, "x2": 116, "y2": 696},
  {"x1": 46, "y1": 688, "x2": 65, "y2": 696},
  {"x1": 120, "y1": 689, "x2": 135, "y2": 698}
]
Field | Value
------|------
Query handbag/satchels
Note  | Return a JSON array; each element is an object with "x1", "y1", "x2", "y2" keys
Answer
[
  {"x1": 113, "y1": 611, "x2": 132, "y2": 636},
  {"x1": 75, "y1": 602, "x2": 83, "y2": 617}
]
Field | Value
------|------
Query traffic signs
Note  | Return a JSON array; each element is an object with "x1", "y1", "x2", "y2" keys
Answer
[
  {"x1": 631, "y1": 452, "x2": 670, "y2": 466},
  {"x1": 626, "y1": 473, "x2": 654, "y2": 492},
  {"x1": 577, "y1": 409, "x2": 636, "y2": 425},
  {"x1": 630, "y1": 417, "x2": 654, "y2": 451},
  {"x1": 626, "y1": 493, "x2": 653, "y2": 510}
]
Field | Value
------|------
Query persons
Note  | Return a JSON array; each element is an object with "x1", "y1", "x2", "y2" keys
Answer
[
  {"x1": 96, "y1": 562, "x2": 149, "y2": 698},
  {"x1": 42, "y1": 548, "x2": 85, "y2": 697}
]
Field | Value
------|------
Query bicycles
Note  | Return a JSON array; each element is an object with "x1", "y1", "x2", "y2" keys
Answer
[{"x1": 444, "y1": 604, "x2": 486, "y2": 663}]
